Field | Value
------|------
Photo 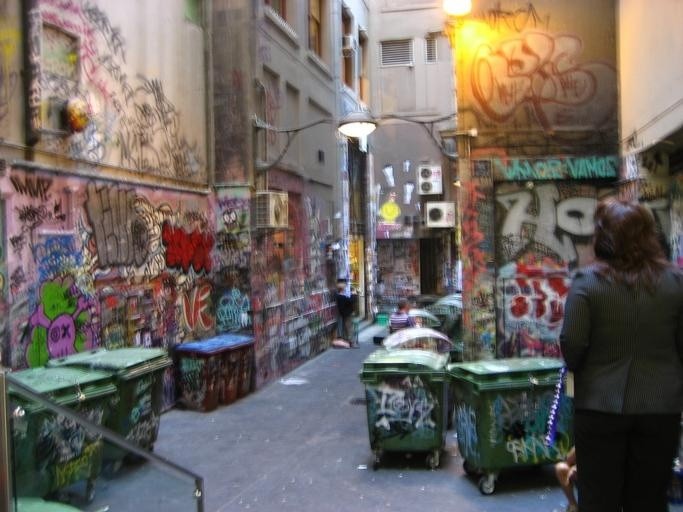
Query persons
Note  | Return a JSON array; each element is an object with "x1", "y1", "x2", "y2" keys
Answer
[
  {"x1": 553, "y1": 444, "x2": 579, "y2": 512},
  {"x1": 389, "y1": 298, "x2": 422, "y2": 335},
  {"x1": 557, "y1": 194, "x2": 681, "y2": 511},
  {"x1": 330, "y1": 282, "x2": 361, "y2": 350}
]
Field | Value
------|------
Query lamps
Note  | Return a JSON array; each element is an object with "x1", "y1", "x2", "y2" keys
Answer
[{"x1": 252, "y1": 111, "x2": 377, "y2": 172}]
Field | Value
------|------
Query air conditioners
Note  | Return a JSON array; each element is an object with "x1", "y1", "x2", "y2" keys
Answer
[
  {"x1": 341, "y1": 34, "x2": 356, "y2": 56},
  {"x1": 424, "y1": 201, "x2": 456, "y2": 229},
  {"x1": 255, "y1": 190, "x2": 289, "y2": 229},
  {"x1": 416, "y1": 165, "x2": 443, "y2": 196}
]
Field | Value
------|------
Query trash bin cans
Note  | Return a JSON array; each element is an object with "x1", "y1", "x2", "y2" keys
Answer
[
  {"x1": 6, "y1": 346, "x2": 173, "y2": 510},
  {"x1": 173, "y1": 334, "x2": 257, "y2": 412},
  {"x1": 446, "y1": 356, "x2": 567, "y2": 495},
  {"x1": 428, "y1": 292, "x2": 467, "y2": 347},
  {"x1": 391, "y1": 309, "x2": 441, "y2": 330},
  {"x1": 383, "y1": 329, "x2": 453, "y2": 427},
  {"x1": 360, "y1": 349, "x2": 451, "y2": 472}
]
